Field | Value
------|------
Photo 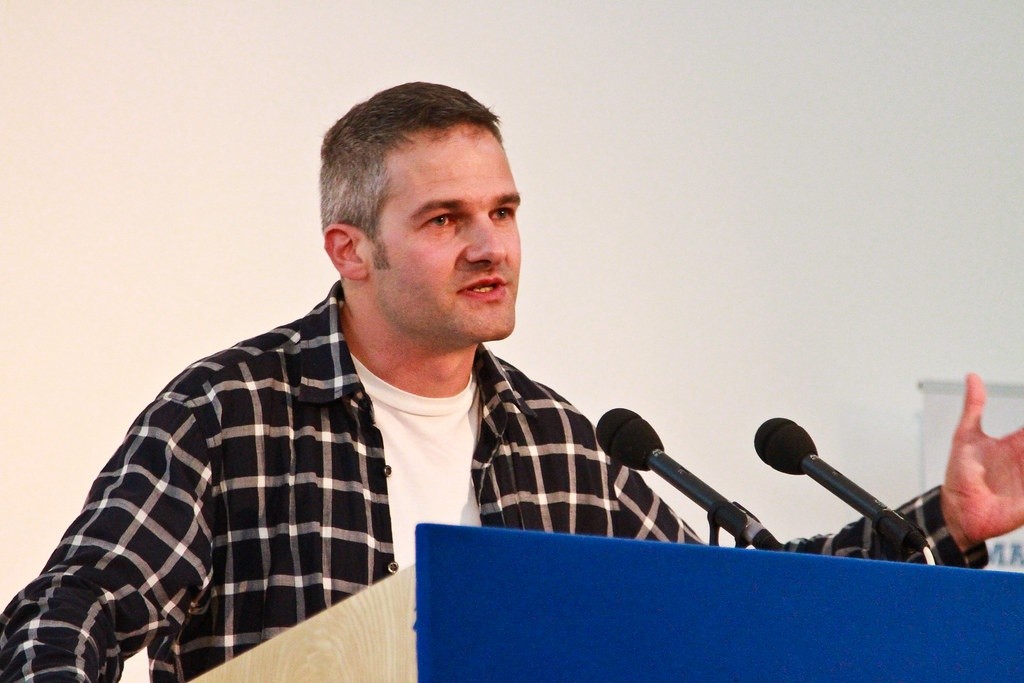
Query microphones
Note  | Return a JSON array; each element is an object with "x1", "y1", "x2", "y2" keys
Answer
[
  {"x1": 596, "y1": 408, "x2": 784, "y2": 551},
  {"x1": 754, "y1": 418, "x2": 931, "y2": 553}
]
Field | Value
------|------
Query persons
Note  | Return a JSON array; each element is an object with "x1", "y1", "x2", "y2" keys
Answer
[{"x1": 0, "y1": 79, "x2": 1023, "y2": 683}]
